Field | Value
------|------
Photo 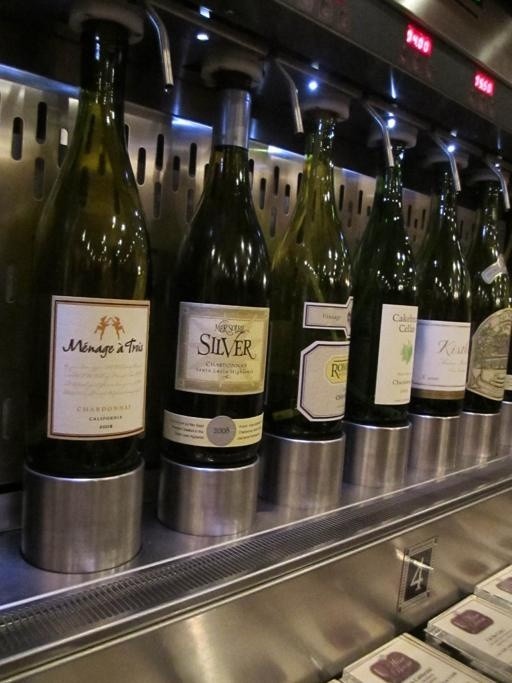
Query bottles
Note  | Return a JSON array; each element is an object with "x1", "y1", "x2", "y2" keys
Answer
[
  {"x1": 469, "y1": 177, "x2": 512, "y2": 413},
  {"x1": 29, "y1": 26, "x2": 144, "y2": 476},
  {"x1": 267, "y1": 115, "x2": 357, "y2": 445},
  {"x1": 353, "y1": 139, "x2": 414, "y2": 430},
  {"x1": 159, "y1": 78, "x2": 272, "y2": 468},
  {"x1": 412, "y1": 159, "x2": 468, "y2": 419}
]
